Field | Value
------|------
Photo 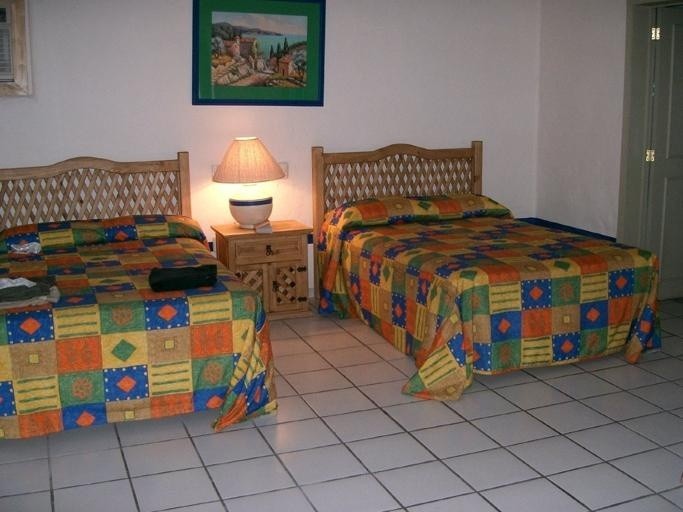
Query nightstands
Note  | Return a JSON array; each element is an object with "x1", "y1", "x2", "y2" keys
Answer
[{"x1": 210, "y1": 220, "x2": 313, "y2": 321}]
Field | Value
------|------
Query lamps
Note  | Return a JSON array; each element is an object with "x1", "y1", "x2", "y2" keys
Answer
[{"x1": 209, "y1": 137, "x2": 285, "y2": 229}]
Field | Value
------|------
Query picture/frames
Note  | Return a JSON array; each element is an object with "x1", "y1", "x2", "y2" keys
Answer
[{"x1": 190, "y1": 1, "x2": 326, "y2": 107}]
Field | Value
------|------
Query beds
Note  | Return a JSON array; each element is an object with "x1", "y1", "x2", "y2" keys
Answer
[
  {"x1": 310, "y1": 139, "x2": 660, "y2": 383},
  {"x1": 0, "y1": 152, "x2": 271, "y2": 438}
]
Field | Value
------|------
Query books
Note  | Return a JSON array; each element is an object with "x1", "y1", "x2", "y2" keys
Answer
[{"x1": 254, "y1": 217, "x2": 272, "y2": 234}]
